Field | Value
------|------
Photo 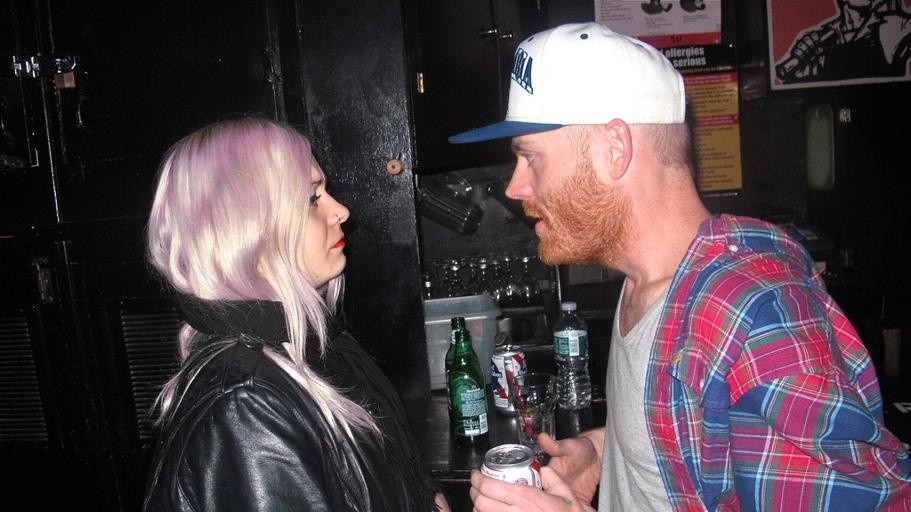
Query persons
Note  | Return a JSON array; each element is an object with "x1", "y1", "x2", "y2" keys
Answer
[
  {"x1": 138, "y1": 110, "x2": 462, "y2": 510},
  {"x1": 774, "y1": 1, "x2": 911, "y2": 86},
  {"x1": 446, "y1": 18, "x2": 911, "y2": 510}
]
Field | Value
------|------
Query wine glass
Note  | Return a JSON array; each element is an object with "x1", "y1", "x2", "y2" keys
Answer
[{"x1": 421, "y1": 250, "x2": 542, "y2": 313}]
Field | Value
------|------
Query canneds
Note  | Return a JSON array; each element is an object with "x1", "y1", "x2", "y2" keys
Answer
[
  {"x1": 491, "y1": 342, "x2": 529, "y2": 414},
  {"x1": 480, "y1": 443, "x2": 543, "y2": 490}
]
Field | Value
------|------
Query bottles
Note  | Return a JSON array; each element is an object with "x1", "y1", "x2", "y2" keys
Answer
[
  {"x1": 443, "y1": 318, "x2": 490, "y2": 439},
  {"x1": 446, "y1": 437, "x2": 492, "y2": 471},
  {"x1": 556, "y1": 411, "x2": 593, "y2": 437},
  {"x1": 551, "y1": 300, "x2": 595, "y2": 409}
]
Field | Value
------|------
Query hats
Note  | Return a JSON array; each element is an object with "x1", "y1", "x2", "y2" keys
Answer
[{"x1": 450, "y1": 21, "x2": 687, "y2": 143}]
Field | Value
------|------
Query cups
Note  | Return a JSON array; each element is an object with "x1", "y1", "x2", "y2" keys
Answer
[{"x1": 513, "y1": 375, "x2": 555, "y2": 444}]
